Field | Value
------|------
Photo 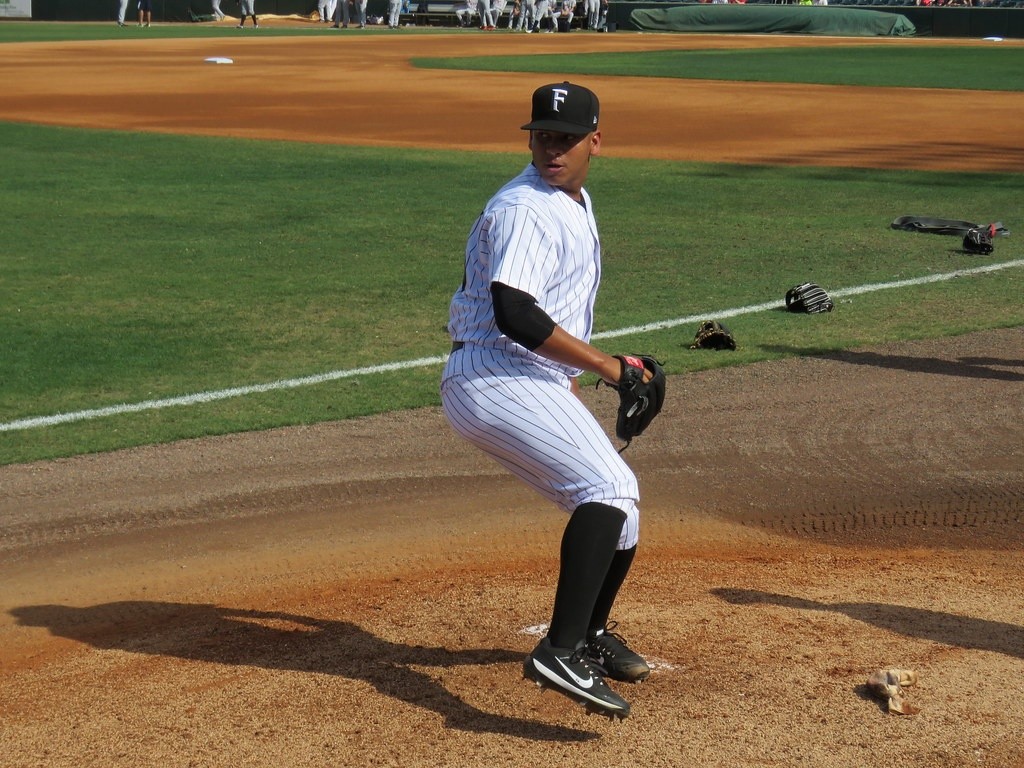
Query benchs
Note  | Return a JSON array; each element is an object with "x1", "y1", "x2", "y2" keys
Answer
[{"x1": 387, "y1": 3, "x2": 588, "y2": 29}]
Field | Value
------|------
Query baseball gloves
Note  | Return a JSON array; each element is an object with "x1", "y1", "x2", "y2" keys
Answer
[
  {"x1": 601, "y1": 355, "x2": 665, "y2": 441},
  {"x1": 964, "y1": 228, "x2": 994, "y2": 254},
  {"x1": 691, "y1": 321, "x2": 736, "y2": 350}
]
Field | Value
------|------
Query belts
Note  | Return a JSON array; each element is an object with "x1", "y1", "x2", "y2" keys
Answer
[{"x1": 450, "y1": 342, "x2": 464, "y2": 354}]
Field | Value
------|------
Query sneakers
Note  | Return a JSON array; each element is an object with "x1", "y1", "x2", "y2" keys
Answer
[
  {"x1": 523, "y1": 637, "x2": 630, "y2": 718},
  {"x1": 586, "y1": 620, "x2": 650, "y2": 682}
]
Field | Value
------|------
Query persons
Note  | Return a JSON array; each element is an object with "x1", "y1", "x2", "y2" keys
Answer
[
  {"x1": 355, "y1": 0, "x2": 367, "y2": 29},
  {"x1": 212, "y1": 0, "x2": 226, "y2": 21},
  {"x1": 117, "y1": 0, "x2": 128, "y2": 27},
  {"x1": 416, "y1": 0, "x2": 433, "y2": 27},
  {"x1": 388, "y1": 0, "x2": 410, "y2": 30},
  {"x1": 456, "y1": 0, "x2": 608, "y2": 33},
  {"x1": 236, "y1": 0, "x2": 258, "y2": 29},
  {"x1": 318, "y1": 0, "x2": 337, "y2": 24},
  {"x1": 699, "y1": 0, "x2": 994, "y2": 8},
  {"x1": 441, "y1": 82, "x2": 665, "y2": 724},
  {"x1": 137, "y1": 0, "x2": 151, "y2": 27},
  {"x1": 330, "y1": 0, "x2": 350, "y2": 28}
]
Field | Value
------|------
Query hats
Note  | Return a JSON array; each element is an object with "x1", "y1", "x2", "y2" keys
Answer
[{"x1": 520, "y1": 81, "x2": 599, "y2": 135}]
